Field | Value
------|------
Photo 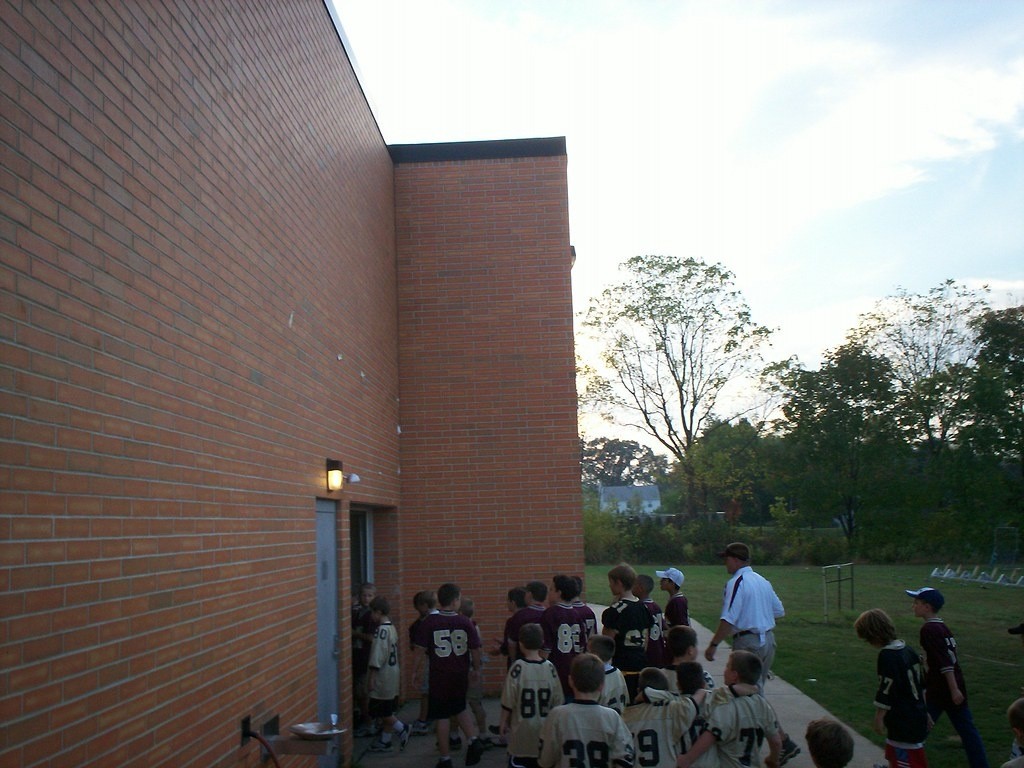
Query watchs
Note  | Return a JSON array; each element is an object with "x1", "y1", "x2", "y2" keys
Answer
[{"x1": 710, "y1": 641, "x2": 718, "y2": 648}]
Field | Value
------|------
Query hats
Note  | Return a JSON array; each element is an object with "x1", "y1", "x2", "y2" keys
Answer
[
  {"x1": 905, "y1": 586, "x2": 944, "y2": 608},
  {"x1": 656, "y1": 567, "x2": 684, "y2": 587},
  {"x1": 716, "y1": 542, "x2": 749, "y2": 559},
  {"x1": 1008, "y1": 623, "x2": 1024, "y2": 635}
]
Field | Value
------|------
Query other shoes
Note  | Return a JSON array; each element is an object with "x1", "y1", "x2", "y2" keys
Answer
[
  {"x1": 872, "y1": 763, "x2": 888, "y2": 768},
  {"x1": 488, "y1": 724, "x2": 501, "y2": 735},
  {"x1": 465, "y1": 739, "x2": 483, "y2": 766},
  {"x1": 435, "y1": 736, "x2": 462, "y2": 748},
  {"x1": 435, "y1": 758, "x2": 452, "y2": 768}
]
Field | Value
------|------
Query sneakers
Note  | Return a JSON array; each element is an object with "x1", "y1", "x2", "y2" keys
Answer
[
  {"x1": 780, "y1": 733, "x2": 800, "y2": 767},
  {"x1": 480, "y1": 738, "x2": 492, "y2": 747},
  {"x1": 411, "y1": 720, "x2": 428, "y2": 732},
  {"x1": 399, "y1": 725, "x2": 412, "y2": 750},
  {"x1": 492, "y1": 737, "x2": 507, "y2": 746},
  {"x1": 370, "y1": 739, "x2": 393, "y2": 751}
]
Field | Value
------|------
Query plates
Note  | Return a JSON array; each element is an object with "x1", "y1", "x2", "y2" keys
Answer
[{"x1": 288, "y1": 723, "x2": 350, "y2": 739}]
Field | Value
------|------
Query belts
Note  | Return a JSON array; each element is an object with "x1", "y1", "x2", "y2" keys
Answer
[{"x1": 733, "y1": 630, "x2": 770, "y2": 639}]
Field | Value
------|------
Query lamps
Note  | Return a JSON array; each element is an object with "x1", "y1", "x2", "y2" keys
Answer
[{"x1": 325, "y1": 455, "x2": 361, "y2": 491}]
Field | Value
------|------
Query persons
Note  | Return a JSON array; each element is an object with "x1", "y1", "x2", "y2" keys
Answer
[
  {"x1": 1000, "y1": 622, "x2": 1024, "y2": 768},
  {"x1": 805, "y1": 720, "x2": 855, "y2": 768},
  {"x1": 408, "y1": 584, "x2": 494, "y2": 768},
  {"x1": 704, "y1": 543, "x2": 801, "y2": 766},
  {"x1": 873, "y1": 586, "x2": 989, "y2": 768},
  {"x1": 487, "y1": 561, "x2": 782, "y2": 768},
  {"x1": 854, "y1": 608, "x2": 935, "y2": 768},
  {"x1": 351, "y1": 582, "x2": 412, "y2": 752}
]
